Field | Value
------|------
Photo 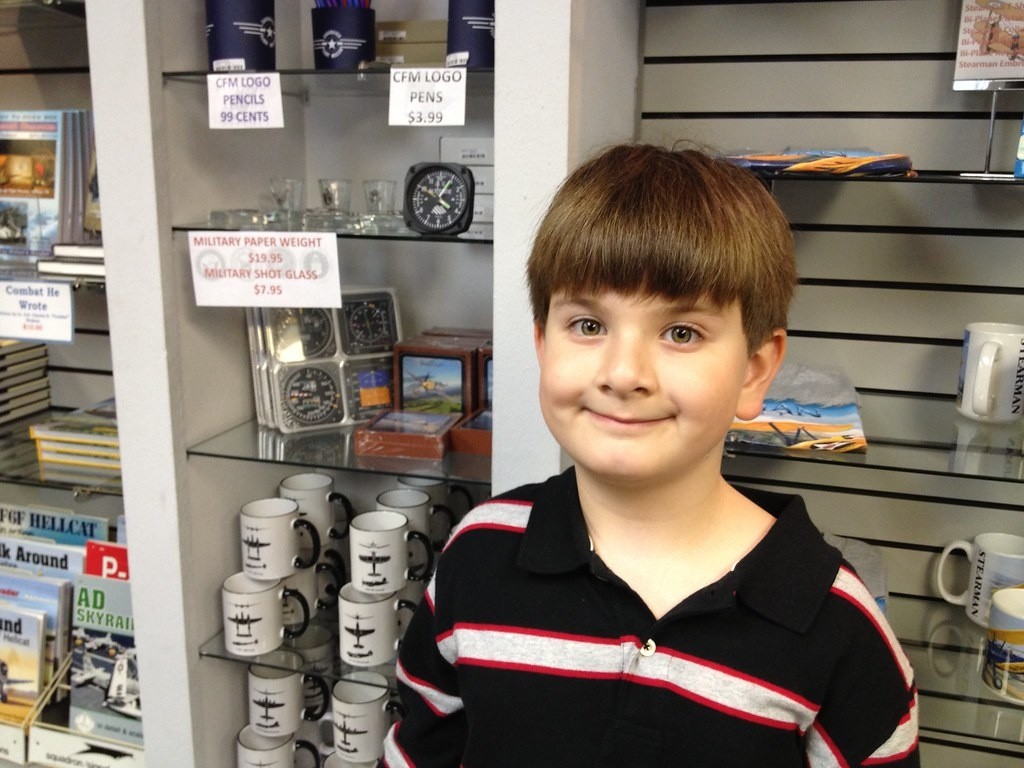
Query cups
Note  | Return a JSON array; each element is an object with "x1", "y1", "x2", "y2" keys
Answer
[
  {"x1": 222, "y1": 572, "x2": 310, "y2": 656},
  {"x1": 317, "y1": 178, "x2": 354, "y2": 213},
  {"x1": 204, "y1": 0, "x2": 276, "y2": 71},
  {"x1": 364, "y1": 180, "x2": 397, "y2": 216},
  {"x1": 240, "y1": 497, "x2": 320, "y2": 580},
  {"x1": 236, "y1": 612, "x2": 406, "y2": 768},
  {"x1": 397, "y1": 476, "x2": 472, "y2": 541},
  {"x1": 956, "y1": 322, "x2": 1024, "y2": 423},
  {"x1": 937, "y1": 532, "x2": 1024, "y2": 628},
  {"x1": 982, "y1": 588, "x2": 1024, "y2": 706},
  {"x1": 349, "y1": 511, "x2": 433, "y2": 594},
  {"x1": 279, "y1": 471, "x2": 354, "y2": 547},
  {"x1": 318, "y1": 541, "x2": 347, "y2": 599},
  {"x1": 446, "y1": 0, "x2": 494, "y2": 67},
  {"x1": 338, "y1": 582, "x2": 418, "y2": 667},
  {"x1": 397, "y1": 579, "x2": 423, "y2": 640},
  {"x1": 378, "y1": 488, "x2": 456, "y2": 552},
  {"x1": 283, "y1": 562, "x2": 341, "y2": 625},
  {"x1": 310, "y1": 8, "x2": 375, "y2": 69},
  {"x1": 270, "y1": 178, "x2": 305, "y2": 211}
]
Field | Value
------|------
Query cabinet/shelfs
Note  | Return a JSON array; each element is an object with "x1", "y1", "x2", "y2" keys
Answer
[
  {"x1": 727, "y1": 166, "x2": 1024, "y2": 709},
  {"x1": 0, "y1": 0, "x2": 145, "y2": 768},
  {"x1": 166, "y1": 58, "x2": 497, "y2": 690}
]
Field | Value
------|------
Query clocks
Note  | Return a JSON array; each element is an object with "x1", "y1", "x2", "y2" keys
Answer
[{"x1": 400, "y1": 161, "x2": 477, "y2": 236}]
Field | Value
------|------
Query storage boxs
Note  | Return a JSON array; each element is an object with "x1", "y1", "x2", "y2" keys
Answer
[
  {"x1": 375, "y1": 18, "x2": 447, "y2": 41},
  {"x1": 375, "y1": 43, "x2": 447, "y2": 64},
  {"x1": 350, "y1": 325, "x2": 493, "y2": 460}
]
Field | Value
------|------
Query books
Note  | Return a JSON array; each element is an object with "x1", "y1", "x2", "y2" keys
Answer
[
  {"x1": 0, "y1": 503, "x2": 143, "y2": 743},
  {"x1": 0, "y1": 108, "x2": 104, "y2": 276},
  {"x1": 0, "y1": 338, "x2": 119, "y2": 471}
]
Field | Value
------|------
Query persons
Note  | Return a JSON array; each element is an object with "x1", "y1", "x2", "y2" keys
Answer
[{"x1": 376, "y1": 145, "x2": 920, "y2": 768}]
